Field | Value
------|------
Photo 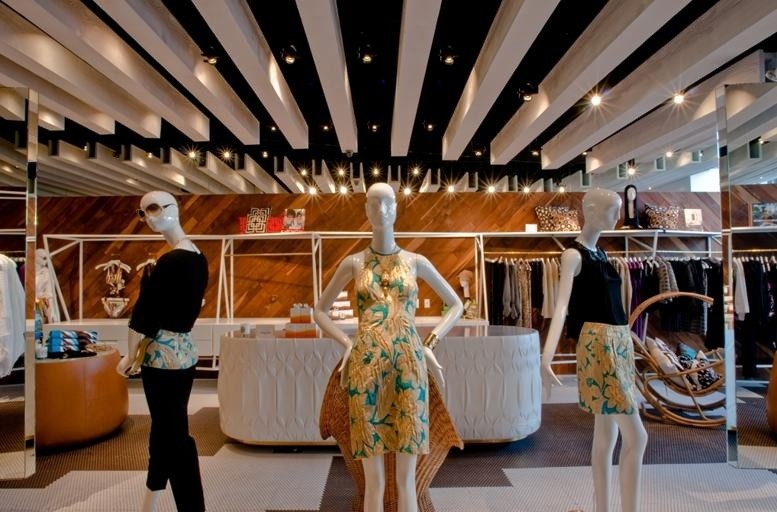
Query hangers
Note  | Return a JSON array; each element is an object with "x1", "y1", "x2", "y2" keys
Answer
[{"x1": 94, "y1": 258, "x2": 156, "y2": 275}]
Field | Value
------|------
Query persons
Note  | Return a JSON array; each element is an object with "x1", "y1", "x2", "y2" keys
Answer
[
  {"x1": 114, "y1": 191, "x2": 208, "y2": 511},
  {"x1": 311, "y1": 181, "x2": 464, "y2": 512},
  {"x1": 623, "y1": 184, "x2": 642, "y2": 231},
  {"x1": 541, "y1": 188, "x2": 648, "y2": 512}
]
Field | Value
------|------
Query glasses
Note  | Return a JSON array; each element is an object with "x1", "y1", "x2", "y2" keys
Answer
[{"x1": 137, "y1": 202, "x2": 177, "y2": 222}]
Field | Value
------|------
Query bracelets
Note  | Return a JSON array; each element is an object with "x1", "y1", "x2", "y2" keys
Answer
[{"x1": 423, "y1": 329, "x2": 438, "y2": 350}]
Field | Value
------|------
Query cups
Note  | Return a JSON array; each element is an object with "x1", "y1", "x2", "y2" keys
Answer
[{"x1": 241, "y1": 323, "x2": 251, "y2": 334}]
